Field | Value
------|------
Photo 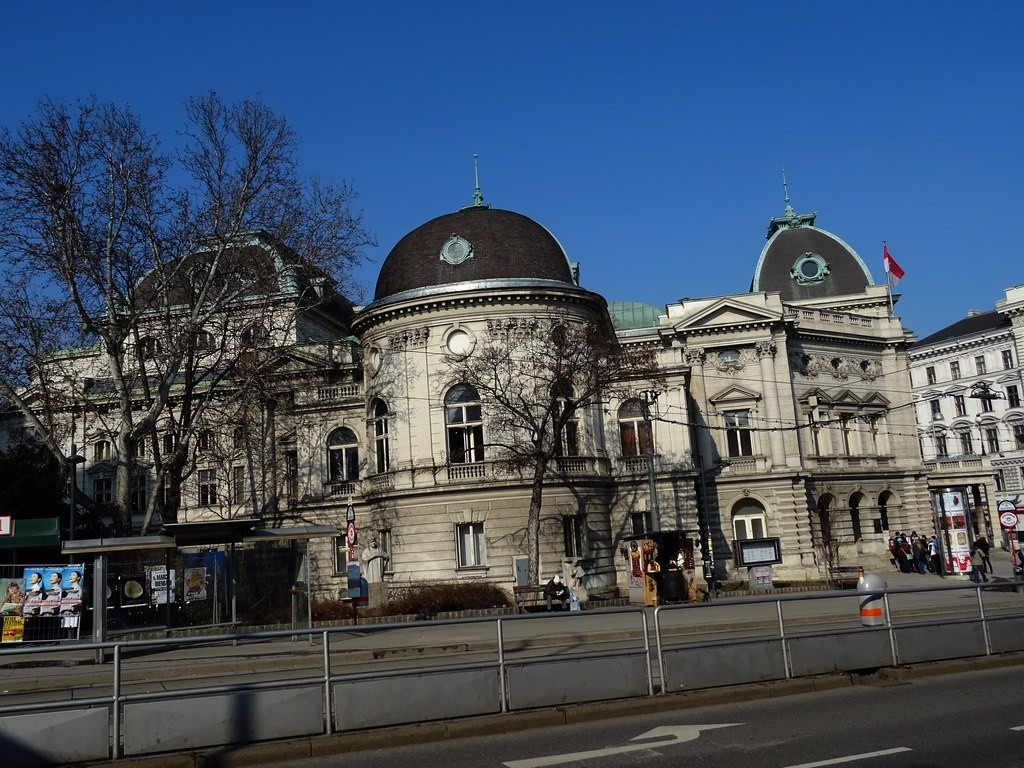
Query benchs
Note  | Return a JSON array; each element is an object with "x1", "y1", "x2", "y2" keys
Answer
[
  {"x1": 828, "y1": 565, "x2": 864, "y2": 589},
  {"x1": 513, "y1": 586, "x2": 570, "y2": 613}
]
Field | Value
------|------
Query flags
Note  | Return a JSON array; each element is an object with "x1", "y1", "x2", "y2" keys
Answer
[{"x1": 884, "y1": 245, "x2": 905, "y2": 288}]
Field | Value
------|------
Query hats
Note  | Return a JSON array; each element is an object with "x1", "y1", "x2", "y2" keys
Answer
[{"x1": 554, "y1": 575, "x2": 560, "y2": 582}]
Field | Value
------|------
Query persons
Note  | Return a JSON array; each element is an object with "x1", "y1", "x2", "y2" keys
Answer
[
  {"x1": 61, "y1": 570, "x2": 81, "y2": 615},
  {"x1": 888, "y1": 531, "x2": 943, "y2": 575},
  {"x1": 40, "y1": 572, "x2": 63, "y2": 616},
  {"x1": 975, "y1": 534, "x2": 994, "y2": 574},
  {"x1": 543, "y1": 574, "x2": 568, "y2": 612},
  {"x1": 25, "y1": 572, "x2": 42, "y2": 615},
  {"x1": 969, "y1": 541, "x2": 990, "y2": 584},
  {"x1": 0, "y1": 582, "x2": 24, "y2": 614}
]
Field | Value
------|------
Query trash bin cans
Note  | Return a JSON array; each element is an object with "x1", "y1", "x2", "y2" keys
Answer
[
  {"x1": 352, "y1": 577, "x2": 369, "y2": 608},
  {"x1": 854, "y1": 574, "x2": 887, "y2": 629}
]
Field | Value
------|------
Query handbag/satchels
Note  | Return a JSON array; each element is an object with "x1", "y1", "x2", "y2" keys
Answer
[{"x1": 906, "y1": 554, "x2": 913, "y2": 560}]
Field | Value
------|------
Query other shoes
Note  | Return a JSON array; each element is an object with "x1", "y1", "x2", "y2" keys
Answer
[
  {"x1": 989, "y1": 571, "x2": 994, "y2": 574},
  {"x1": 919, "y1": 571, "x2": 926, "y2": 574},
  {"x1": 975, "y1": 581, "x2": 980, "y2": 583},
  {"x1": 984, "y1": 571, "x2": 987, "y2": 573},
  {"x1": 562, "y1": 608, "x2": 568, "y2": 611},
  {"x1": 548, "y1": 609, "x2": 552, "y2": 612},
  {"x1": 984, "y1": 579, "x2": 989, "y2": 582}
]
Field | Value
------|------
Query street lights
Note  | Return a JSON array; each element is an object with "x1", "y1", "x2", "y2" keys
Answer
[
  {"x1": 64, "y1": 443, "x2": 87, "y2": 563},
  {"x1": 638, "y1": 390, "x2": 664, "y2": 532}
]
